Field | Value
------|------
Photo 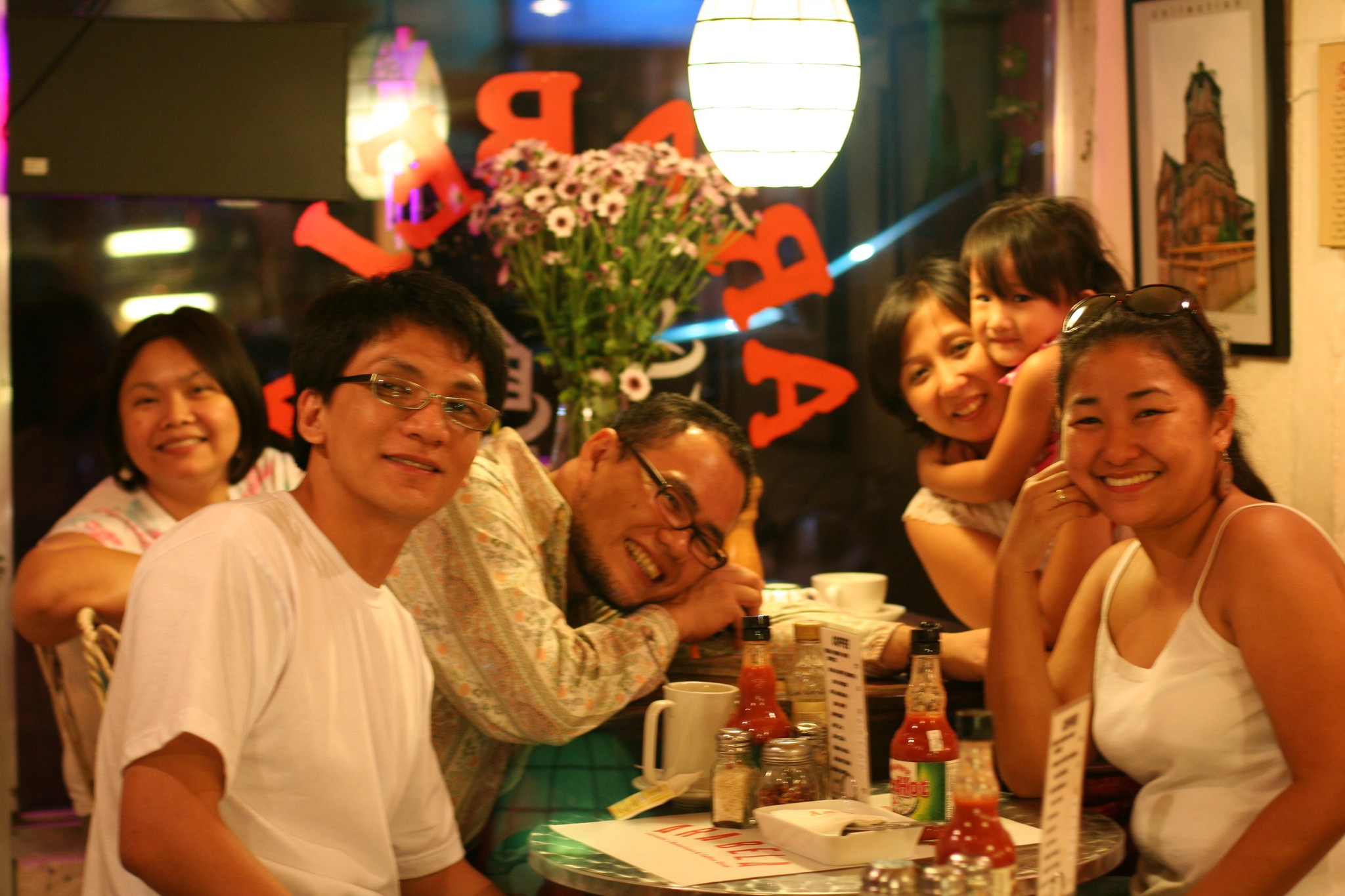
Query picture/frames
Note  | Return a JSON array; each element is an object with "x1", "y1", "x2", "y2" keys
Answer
[{"x1": 1123, "y1": 0, "x2": 1294, "y2": 363}]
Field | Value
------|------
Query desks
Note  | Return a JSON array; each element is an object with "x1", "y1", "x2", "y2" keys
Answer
[{"x1": 524, "y1": 790, "x2": 1125, "y2": 896}]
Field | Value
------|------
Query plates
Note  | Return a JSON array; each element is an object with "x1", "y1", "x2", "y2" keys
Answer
[
  {"x1": 806, "y1": 604, "x2": 907, "y2": 622},
  {"x1": 752, "y1": 795, "x2": 927, "y2": 865},
  {"x1": 631, "y1": 775, "x2": 713, "y2": 808}
]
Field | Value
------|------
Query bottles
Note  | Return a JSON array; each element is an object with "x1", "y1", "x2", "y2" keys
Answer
[
  {"x1": 795, "y1": 723, "x2": 835, "y2": 800},
  {"x1": 725, "y1": 615, "x2": 793, "y2": 766},
  {"x1": 936, "y1": 710, "x2": 1018, "y2": 896},
  {"x1": 710, "y1": 728, "x2": 760, "y2": 829},
  {"x1": 785, "y1": 620, "x2": 830, "y2": 799},
  {"x1": 755, "y1": 738, "x2": 818, "y2": 806},
  {"x1": 888, "y1": 622, "x2": 962, "y2": 824}
]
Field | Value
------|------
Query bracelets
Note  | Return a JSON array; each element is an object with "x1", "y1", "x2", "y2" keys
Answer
[{"x1": 910, "y1": 620, "x2": 943, "y2": 654}]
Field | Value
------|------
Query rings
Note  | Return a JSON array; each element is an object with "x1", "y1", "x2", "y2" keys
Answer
[{"x1": 1055, "y1": 489, "x2": 1066, "y2": 503}]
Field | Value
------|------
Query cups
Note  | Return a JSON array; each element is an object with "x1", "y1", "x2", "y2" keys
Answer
[
  {"x1": 799, "y1": 572, "x2": 889, "y2": 613},
  {"x1": 643, "y1": 680, "x2": 740, "y2": 796}
]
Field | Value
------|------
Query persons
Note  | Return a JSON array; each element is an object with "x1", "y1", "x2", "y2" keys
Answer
[
  {"x1": 85, "y1": 273, "x2": 512, "y2": 896},
  {"x1": 386, "y1": 392, "x2": 984, "y2": 840},
  {"x1": 9, "y1": 305, "x2": 307, "y2": 645},
  {"x1": 868, "y1": 257, "x2": 1136, "y2": 651},
  {"x1": 982, "y1": 282, "x2": 1345, "y2": 896},
  {"x1": 915, "y1": 197, "x2": 1131, "y2": 504}
]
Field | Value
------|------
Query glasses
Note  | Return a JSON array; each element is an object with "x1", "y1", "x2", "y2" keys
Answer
[
  {"x1": 283, "y1": 373, "x2": 501, "y2": 433},
  {"x1": 1061, "y1": 283, "x2": 1215, "y2": 347},
  {"x1": 617, "y1": 436, "x2": 728, "y2": 570}
]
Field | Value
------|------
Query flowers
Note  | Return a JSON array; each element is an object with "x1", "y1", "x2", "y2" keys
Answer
[{"x1": 470, "y1": 128, "x2": 758, "y2": 462}]
